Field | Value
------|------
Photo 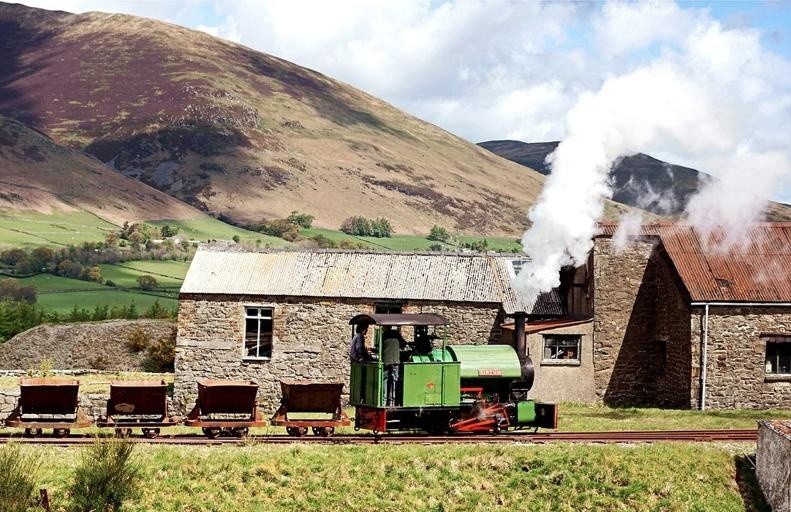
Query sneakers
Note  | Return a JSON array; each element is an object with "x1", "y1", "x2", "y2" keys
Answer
[{"x1": 360, "y1": 399, "x2": 402, "y2": 407}]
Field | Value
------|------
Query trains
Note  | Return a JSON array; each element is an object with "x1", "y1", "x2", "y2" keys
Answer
[{"x1": 0, "y1": 313, "x2": 559, "y2": 440}]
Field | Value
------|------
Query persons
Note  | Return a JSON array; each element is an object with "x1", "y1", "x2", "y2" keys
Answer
[
  {"x1": 349, "y1": 321, "x2": 378, "y2": 362},
  {"x1": 408, "y1": 328, "x2": 434, "y2": 356},
  {"x1": 374, "y1": 324, "x2": 408, "y2": 407}
]
